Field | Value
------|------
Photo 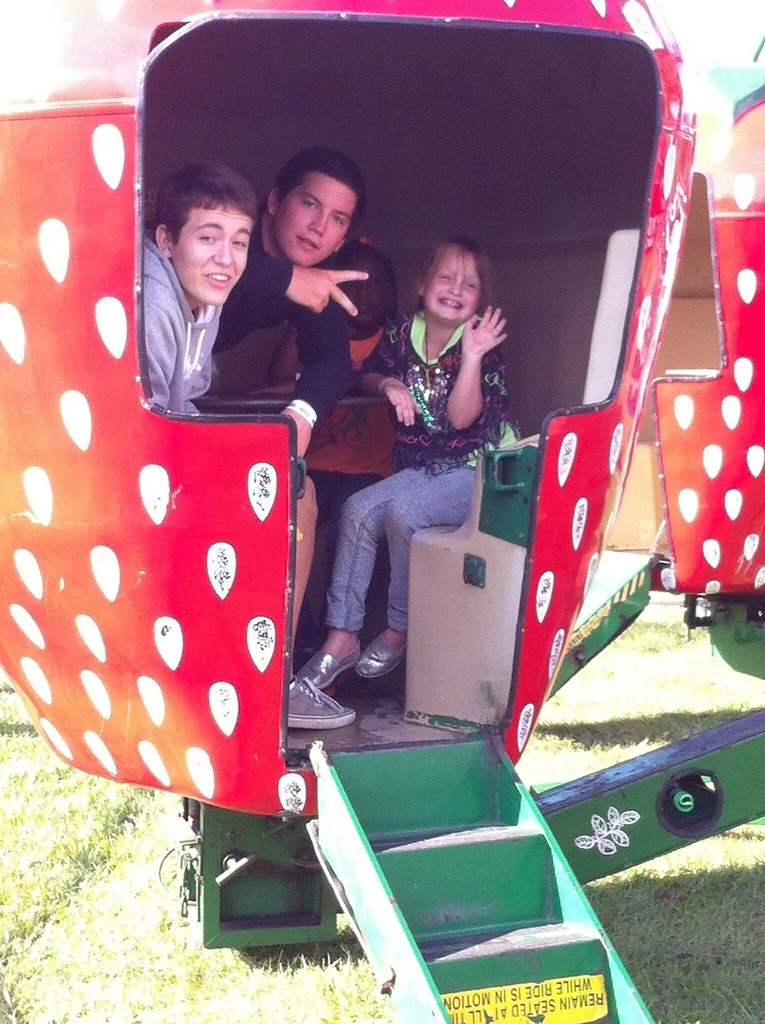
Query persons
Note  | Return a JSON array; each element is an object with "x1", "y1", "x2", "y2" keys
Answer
[
  {"x1": 144, "y1": 148, "x2": 395, "y2": 730},
  {"x1": 296, "y1": 235, "x2": 528, "y2": 690}
]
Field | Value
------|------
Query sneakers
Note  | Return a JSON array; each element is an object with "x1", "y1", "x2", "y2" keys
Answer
[{"x1": 287, "y1": 675, "x2": 356, "y2": 729}]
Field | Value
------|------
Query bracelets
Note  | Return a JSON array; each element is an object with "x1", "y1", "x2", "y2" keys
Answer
[
  {"x1": 286, "y1": 399, "x2": 317, "y2": 428},
  {"x1": 379, "y1": 377, "x2": 407, "y2": 396}
]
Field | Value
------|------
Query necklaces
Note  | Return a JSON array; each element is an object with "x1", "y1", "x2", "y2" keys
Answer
[{"x1": 426, "y1": 329, "x2": 450, "y2": 388}]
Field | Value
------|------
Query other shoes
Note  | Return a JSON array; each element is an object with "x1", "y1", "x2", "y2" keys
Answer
[
  {"x1": 297, "y1": 637, "x2": 361, "y2": 689},
  {"x1": 355, "y1": 634, "x2": 409, "y2": 678}
]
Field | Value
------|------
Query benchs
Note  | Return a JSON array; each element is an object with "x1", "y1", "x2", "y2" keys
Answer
[{"x1": 403, "y1": 432, "x2": 538, "y2": 732}]
